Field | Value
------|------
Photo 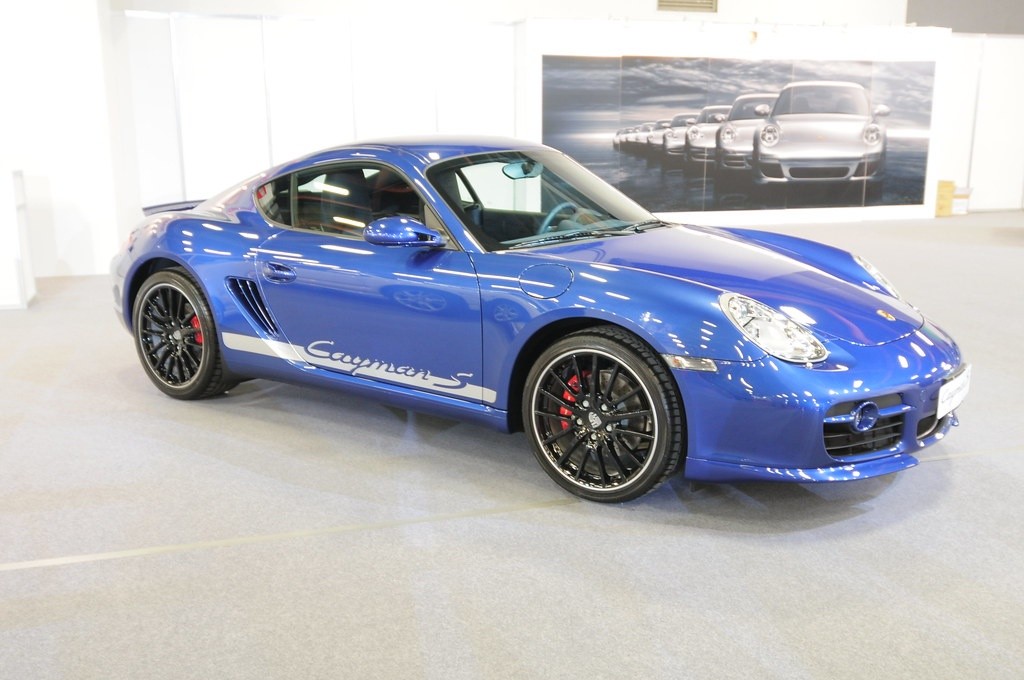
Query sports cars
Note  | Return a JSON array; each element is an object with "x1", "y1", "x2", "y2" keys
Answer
[{"x1": 112, "y1": 140, "x2": 971, "y2": 505}]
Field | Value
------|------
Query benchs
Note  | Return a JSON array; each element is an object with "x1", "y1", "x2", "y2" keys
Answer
[{"x1": 273, "y1": 190, "x2": 322, "y2": 232}]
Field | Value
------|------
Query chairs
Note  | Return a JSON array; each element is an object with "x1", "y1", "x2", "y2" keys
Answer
[
  {"x1": 790, "y1": 97, "x2": 810, "y2": 115},
  {"x1": 376, "y1": 169, "x2": 501, "y2": 250},
  {"x1": 318, "y1": 169, "x2": 372, "y2": 238}
]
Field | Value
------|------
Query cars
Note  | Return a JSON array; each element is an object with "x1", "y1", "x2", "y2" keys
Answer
[
  {"x1": 753, "y1": 81, "x2": 887, "y2": 194},
  {"x1": 613, "y1": 126, "x2": 638, "y2": 145},
  {"x1": 635, "y1": 123, "x2": 655, "y2": 145},
  {"x1": 684, "y1": 106, "x2": 731, "y2": 166},
  {"x1": 714, "y1": 92, "x2": 781, "y2": 178},
  {"x1": 664, "y1": 113, "x2": 699, "y2": 157},
  {"x1": 648, "y1": 119, "x2": 673, "y2": 148}
]
